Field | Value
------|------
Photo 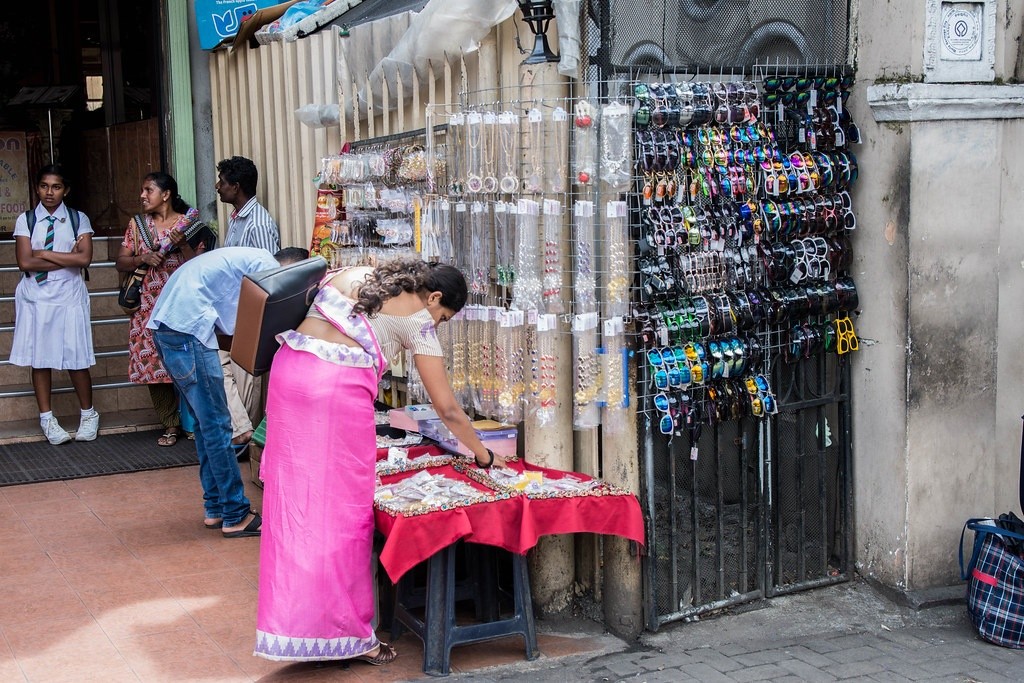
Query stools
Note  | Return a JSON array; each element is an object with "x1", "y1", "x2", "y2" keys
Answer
[{"x1": 384, "y1": 537, "x2": 541, "y2": 678}]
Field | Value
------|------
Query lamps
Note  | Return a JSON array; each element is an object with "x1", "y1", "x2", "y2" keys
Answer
[{"x1": 81, "y1": 28, "x2": 102, "y2": 67}]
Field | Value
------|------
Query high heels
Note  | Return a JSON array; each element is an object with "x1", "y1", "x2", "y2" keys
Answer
[{"x1": 343, "y1": 644, "x2": 397, "y2": 668}]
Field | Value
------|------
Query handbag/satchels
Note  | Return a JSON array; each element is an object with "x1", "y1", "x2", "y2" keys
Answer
[
  {"x1": 959, "y1": 518, "x2": 1024, "y2": 649},
  {"x1": 230, "y1": 254, "x2": 353, "y2": 377},
  {"x1": 118, "y1": 218, "x2": 148, "y2": 315}
]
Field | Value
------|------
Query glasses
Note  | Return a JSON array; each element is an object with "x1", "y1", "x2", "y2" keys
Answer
[{"x1": 633, "y1": 75, "x2": 862, "y2": 447}]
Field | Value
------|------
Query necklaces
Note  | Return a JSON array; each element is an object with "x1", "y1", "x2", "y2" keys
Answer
[{"x1": 415, "y1": 97, "x2": 628, "y2": 432}]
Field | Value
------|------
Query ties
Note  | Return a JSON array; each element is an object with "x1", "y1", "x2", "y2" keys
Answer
[{"x1": 34, "y1": 216, "x2": 57, "y2": 285}]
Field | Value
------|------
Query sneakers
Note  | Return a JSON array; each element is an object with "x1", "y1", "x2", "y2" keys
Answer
[
  {"x1": 75, "y1": 411, "x2": 99, "y2": 441},
  {"x1": 40, "y1": 417, "x2": 71, "y2": 445}
]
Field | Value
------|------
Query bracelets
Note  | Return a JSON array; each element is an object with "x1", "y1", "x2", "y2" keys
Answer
[
  {"x1": 132, "y1": 255, "x2": 142, "y2": 266},
  {"x1": 475, "y1": 448, "x2": 494, "y2": 469},
  {"x1": 319, "y1": 138, "x2": 427, "y2": 265}
]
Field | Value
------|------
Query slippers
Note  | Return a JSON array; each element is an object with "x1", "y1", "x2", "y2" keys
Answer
[
  {"x1": 222, "y1": 509, "x2": 262, "y2": 538},
  {"x1": 206, "y1": 520, "x2": 222, "y2": 529},
  {"x1": 158, "y1": 433, "x2": 177, "y2": 446},
  {"x1": 187, "y1": 434, "x2": 195, "y2": 440}
]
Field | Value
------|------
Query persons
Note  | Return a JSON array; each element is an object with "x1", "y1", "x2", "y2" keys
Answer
[
  {"x1": 116, "y1": 171, "x2": 213, "y2": 446},
  {"x1": 210, "y1": 158, "x2": 283, "y2": 462},
  {"x1": 9, "y1": 169, "x2": 100, "y2": 443},
  {"x1": 253, "y1": 257, "x2": 510, "y2": 666},
  {"x1": 145, "y1": 246, "x2": 315, "y2": 540}
]
